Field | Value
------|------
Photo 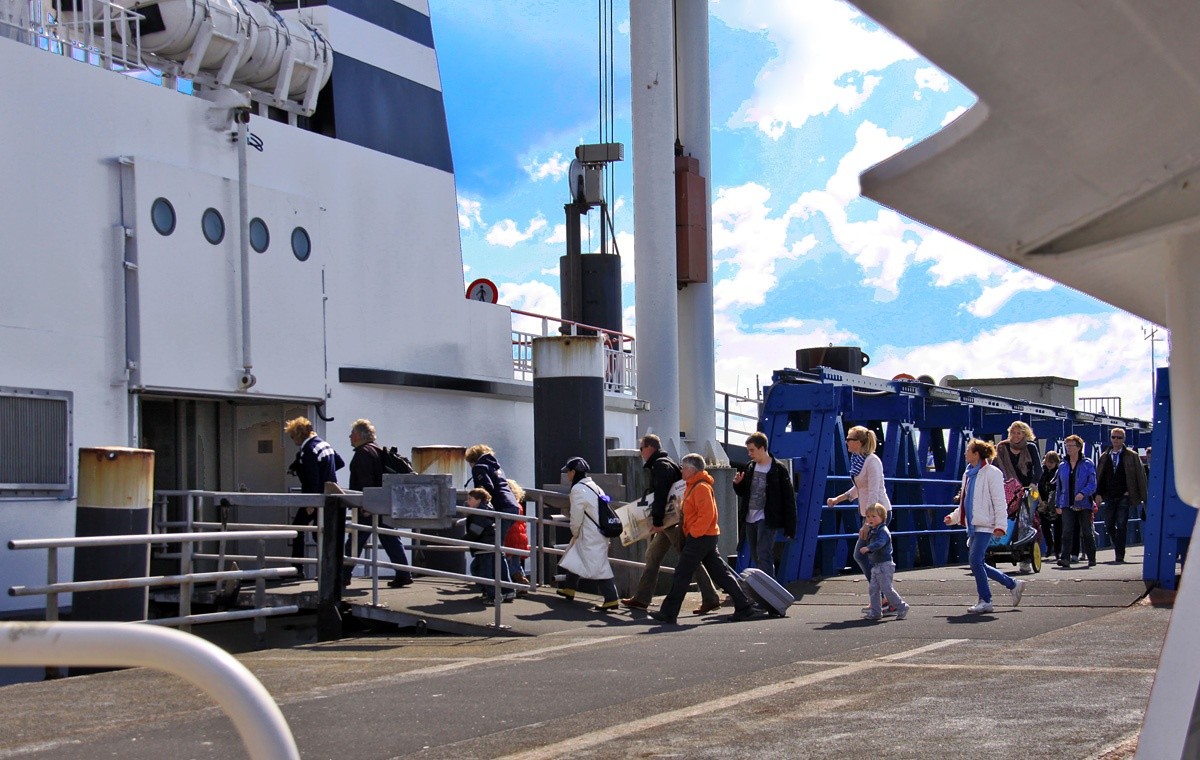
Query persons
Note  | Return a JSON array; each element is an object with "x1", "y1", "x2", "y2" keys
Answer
[
  {"x1": 461, "y1": 445, "x2": 532, "y2": 605},
  {"x1": 825, "y1": 426, "x2": 912, "y2": 622},
  {"x1": 559, "y1": 458, "x2": 621, "y2": 611},
  {"x1": 334, "y1": 419, "x2": 414, "y2": 589},
  {"x1": 943, "y1": 420, "x2": 1150, "y2": 614},
  {"x1": 645, "y1": 454, "x2": 757, "y2": 624},
  {"x1": 284, "y1": 417, "x2": 344, "y2": 579},
  {"x1": 731, "y1": 433, "x2": 799, "y2": 615},
  {"x1": 621, "y1": 434, "x2": 722, "y2": 614}
]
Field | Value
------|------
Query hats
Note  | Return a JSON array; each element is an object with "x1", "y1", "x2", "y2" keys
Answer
[{"x1": 561, "y1": 457, "x2": 590, "y2": 473}]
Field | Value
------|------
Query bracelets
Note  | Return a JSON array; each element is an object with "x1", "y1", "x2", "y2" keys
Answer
[{"x1": 1079, "y1": 491, "x2": 1085, "y2": 495}]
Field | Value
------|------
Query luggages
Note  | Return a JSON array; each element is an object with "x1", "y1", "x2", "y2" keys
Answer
[{"x1": 727, "y1": 563, "x2": 795, "y2": 617}]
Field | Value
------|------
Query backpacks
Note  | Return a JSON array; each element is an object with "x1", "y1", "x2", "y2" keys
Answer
[
  {"x1": 367, "y1": 442, "x2": 413, "y2": 473},
  {"x1": 577, "y1": 481, "x2": 623, "y2": 538}
]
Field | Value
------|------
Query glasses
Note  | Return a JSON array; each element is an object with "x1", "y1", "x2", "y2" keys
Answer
[
  {"x1": 846, "y1": 436, "x2": 858, "y2": 442},
  {"x1": 640, "y1": 447, "x2": 647, "y2": 451},
  {"x1": 1112, "y1": 435, "x2": 1123, "y2": 440},
  {"x1": 1065, "y1": 444, "x2": 1078, "y2": 448}
]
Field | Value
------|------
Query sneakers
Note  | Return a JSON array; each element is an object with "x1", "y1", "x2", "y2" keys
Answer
[
  {"x1": 967, "y1": 600, "x2": 993, "y2": 614},
  {"x1": 1010, "y1": 580, "x2": 1025, "y2": 607},
  {"x1": 861, "y1": 601, "x2": 910, "y2": 620}
]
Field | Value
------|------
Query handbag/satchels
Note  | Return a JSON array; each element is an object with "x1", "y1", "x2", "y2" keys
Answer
[
  {"x1": 673, "y1": 513, "x2": 686, "y2": 553},
  {"x1": 988, "y1": 478, "x2": 1024, "y2": 548}
]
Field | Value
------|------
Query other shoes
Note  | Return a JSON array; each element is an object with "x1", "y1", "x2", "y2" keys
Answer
[
  {"x1": 1019, "y1": 551, "x2": 1125, "y2": 573},
  {"x1": 595, "y1": 600, "x2": 619, "y2": 610},
  {"x1": 647, "y1": 610, "x2": 677, "y2": 625},
  {"x1": 480, "y1": 576, "x2": 530, "y2": 605},
  {"x1": 693, "y1": 603, "x2": 721, "y2": 615},
  {"x1": 344, "y1": 578, "x2": 351, "y2": 585},
  {"x1": 290, "y1": 571, "x2": 305, "y2": 580},
  {"x1": 556, "y1": 588, "x2": 575, "y2": 601},
  {"x1": 621, "y1": 598, "x2": 648, "y2": 611},
  {"x1": 727, "y1": 608, "x2": 756, "y2": 621},
  {"x1": 388, "y1": 577, "x2": 414, "y2": 586}
]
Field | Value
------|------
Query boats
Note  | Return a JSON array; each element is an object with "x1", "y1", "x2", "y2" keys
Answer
[{"x1": 0, "y1": 0, "x2": 769, "y2": 689}]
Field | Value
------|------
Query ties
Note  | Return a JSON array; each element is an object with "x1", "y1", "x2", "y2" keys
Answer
[{"x1": 1112, "y1": 452, "x2": 1119, "y2": 470}]
